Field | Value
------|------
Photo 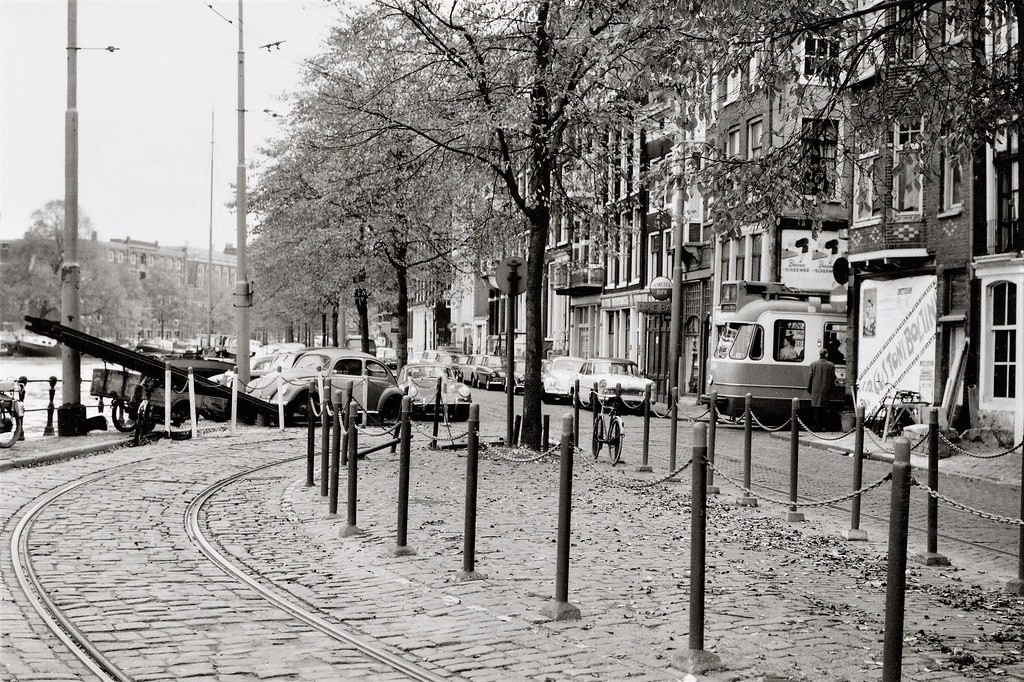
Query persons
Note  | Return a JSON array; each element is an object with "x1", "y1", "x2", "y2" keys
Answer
[
  {"x1": 780, "y1": 335, "x2": 798, "y2": 360},
  {"x1": 829, "y1": 339, "x2": 844, "y2": 363},
  {"x1": 798, "y1": 349, "x2": 804, "y2": 359},
  {"x1": 807, "y1": 349, "x2": 837, "y2": 432}
]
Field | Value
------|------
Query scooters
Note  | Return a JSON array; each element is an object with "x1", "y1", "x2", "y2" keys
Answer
[{"x1": 0, "y1": 379, "x2": 26, "y2": 448}]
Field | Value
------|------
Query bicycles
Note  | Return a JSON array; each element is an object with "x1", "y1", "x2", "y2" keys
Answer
[
  {"x1": 863, "y1": 382, "x2": 921, "y2": 439},
  {"x1": 130, "y1": 376, "x2": 163, "y2": 447},
  {"x1": 587, "y1": 389, "x2": 626, "y2": 467}
]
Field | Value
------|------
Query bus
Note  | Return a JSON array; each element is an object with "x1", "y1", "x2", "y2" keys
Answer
[{"x1": 699, "y1": 280, "x2": 847, "y2": 432}]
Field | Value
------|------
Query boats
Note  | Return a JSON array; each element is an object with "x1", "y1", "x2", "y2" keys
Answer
[{"x1": 0, "y1": 328, "x2": 63, "y2": 359}]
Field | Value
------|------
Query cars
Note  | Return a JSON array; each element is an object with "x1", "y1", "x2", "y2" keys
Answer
[
  {"x1": 503, "y1": 359, "x2": 525, "y2": 396},
  {"x1": 245, "y1": 347, "x2": 404, "y2": 427},
  {"x1": 570, "y1": 357, "x2": 658, "y2": 416},
  {"x1": 120, "y1": 333, "x2": 396, "y2": 376},
  {"x1": 420, "y1": 348, "x2": 506, "y2": 391},
  {"x1": 541, "y1": 355, "x2": 586, "y2": 405},
  {"x1": 395, "y1": 360, "x2": 472, "y2": 422}
]
